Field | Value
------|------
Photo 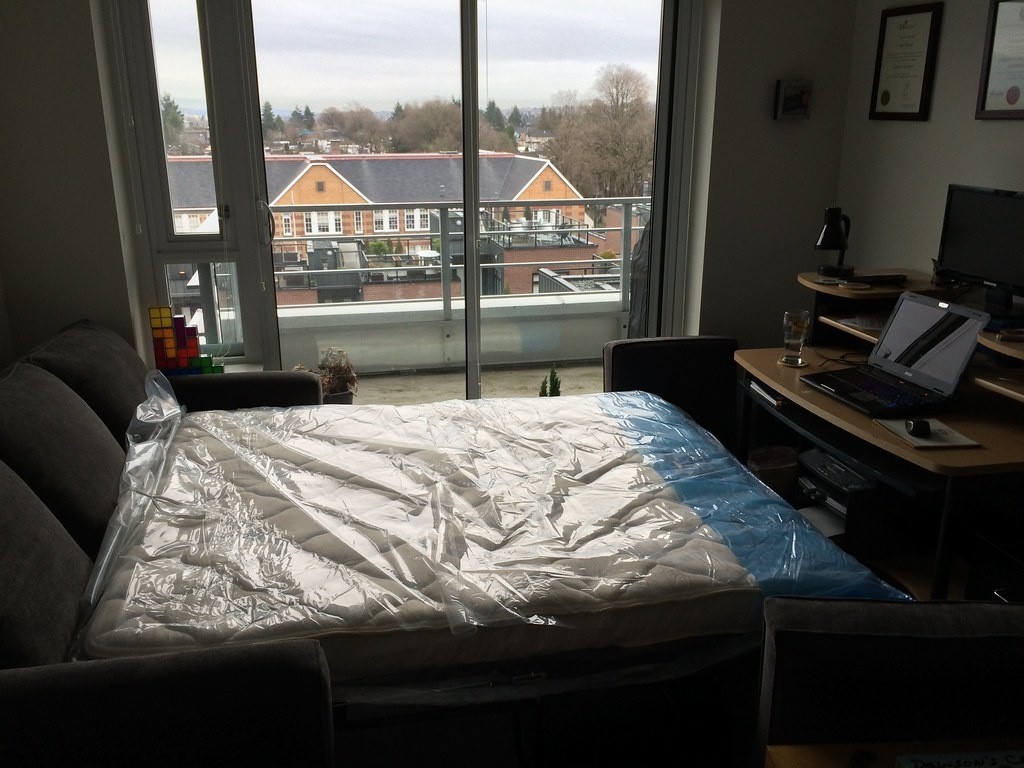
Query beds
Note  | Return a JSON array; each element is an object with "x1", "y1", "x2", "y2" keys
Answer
[{"x1": 0, "y1": 371, "x2": 911, "y2": 768}]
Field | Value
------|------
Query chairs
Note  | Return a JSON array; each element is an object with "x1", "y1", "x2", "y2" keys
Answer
[{"x1": 603, "y1": 336, "x2": 738, "y2": 439}]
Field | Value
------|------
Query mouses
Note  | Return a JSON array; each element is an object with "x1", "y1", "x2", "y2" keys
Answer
[{"x1": 905, "y1": 420, "x2": 931, "y2": 438}]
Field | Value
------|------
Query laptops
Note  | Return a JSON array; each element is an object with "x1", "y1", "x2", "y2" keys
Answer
[{"x1": 798, "y1": 290, "x2": 991, "y2": 418}]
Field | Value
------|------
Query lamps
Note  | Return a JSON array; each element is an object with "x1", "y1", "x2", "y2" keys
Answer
[{"x1": 813, "y1": 206, "x2": 855, "y2": 277}]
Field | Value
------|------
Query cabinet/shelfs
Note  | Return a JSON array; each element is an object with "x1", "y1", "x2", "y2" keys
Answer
[{"x1": 727, "y1": 267, "x2": 1024, "y2": 561}]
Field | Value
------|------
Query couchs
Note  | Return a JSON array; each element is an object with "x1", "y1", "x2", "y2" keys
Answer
[{"x1": 755, "y1": 596, "x2": 1024, "y2": 768}]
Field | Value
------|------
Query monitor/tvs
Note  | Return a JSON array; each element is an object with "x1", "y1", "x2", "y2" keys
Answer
[{"x1": 935, "y1": 184, "x2": 1024, "y2": 334}]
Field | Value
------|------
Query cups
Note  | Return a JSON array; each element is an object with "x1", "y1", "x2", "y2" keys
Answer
[{"x1": 782, "y1": 308, "x2": 811, "y2": 364}]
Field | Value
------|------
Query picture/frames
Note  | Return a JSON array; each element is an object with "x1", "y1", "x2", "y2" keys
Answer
[
  {"x1": 869, "y1": 2, "x2": 944, "y2": 121},
  {"x1": 975, "y1": 0, "x2": 1024, "y2": 120}
]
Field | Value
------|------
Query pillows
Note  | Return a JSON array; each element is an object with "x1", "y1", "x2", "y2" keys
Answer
[
  {"x1": 1, "y1": 461, "x2": 95, "y2": 683},
  {"x1": 1, "y1": 362, "x2": 127, "y2": 552},
  {"x1": 22, "y1": 317, "x2": 150, "y2": 446}
]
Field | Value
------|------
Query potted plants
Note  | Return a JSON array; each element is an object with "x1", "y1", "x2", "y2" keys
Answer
[{"x1": 292, "y1": 346, "x2": 360, "y2": 405}]
